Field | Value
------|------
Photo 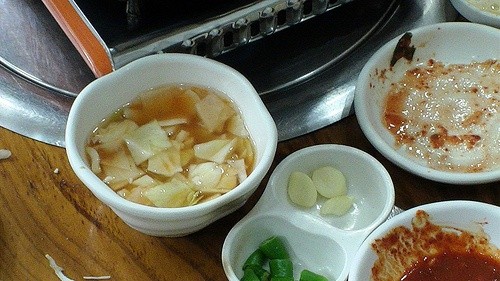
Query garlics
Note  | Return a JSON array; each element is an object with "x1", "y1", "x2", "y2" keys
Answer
[{"x1": 287, "y1": 167, "x2": 356, "y2": 216}]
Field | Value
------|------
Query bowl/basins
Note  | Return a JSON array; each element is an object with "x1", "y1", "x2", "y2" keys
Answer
[
  {"x1": 354, "y1": 21, "x2": 500, "y2": 185},
  {"x1": 222, "y1": 142, "x2": 395, "y2": 280},
  {"x1": 348, "y1": 200, "x2": 500, "y2": 281},
  {"x1": 451, "y1": 0, "x2": 500, "y2": 29},
  {"x1": 65, "y1": 53, "x2": 279, "y2": 237}
]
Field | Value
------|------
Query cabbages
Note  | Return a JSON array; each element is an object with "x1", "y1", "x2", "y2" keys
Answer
[{"x1": 88, "y1": 84, "x2": 254, "y2": 208}]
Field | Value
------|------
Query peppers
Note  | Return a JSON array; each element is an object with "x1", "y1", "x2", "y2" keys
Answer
[{"x1": 242, "y1": 236, "x2": 327, "y2": 281}]
